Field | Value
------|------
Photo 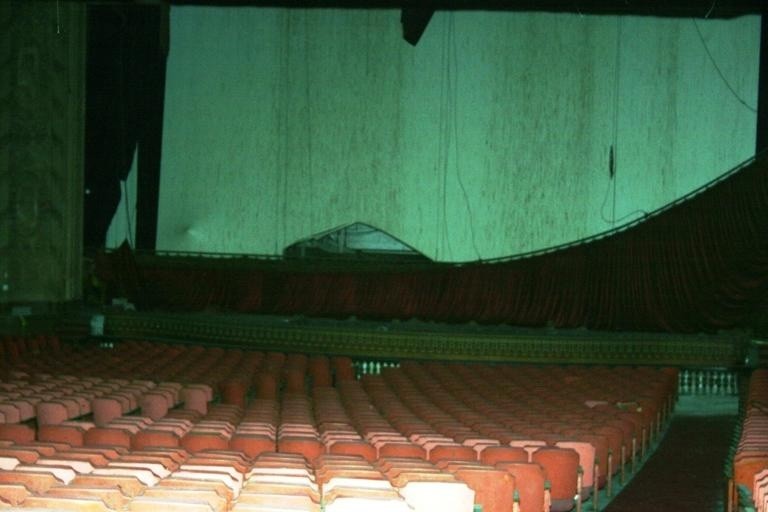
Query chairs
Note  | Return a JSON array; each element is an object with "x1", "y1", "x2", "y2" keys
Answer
[
  {"x1": 0, "y1": 335, "x2": 679, "y2": 511},
  {"x1": 719, "y1": 357, "x2": 767, "y2": 512}
]
